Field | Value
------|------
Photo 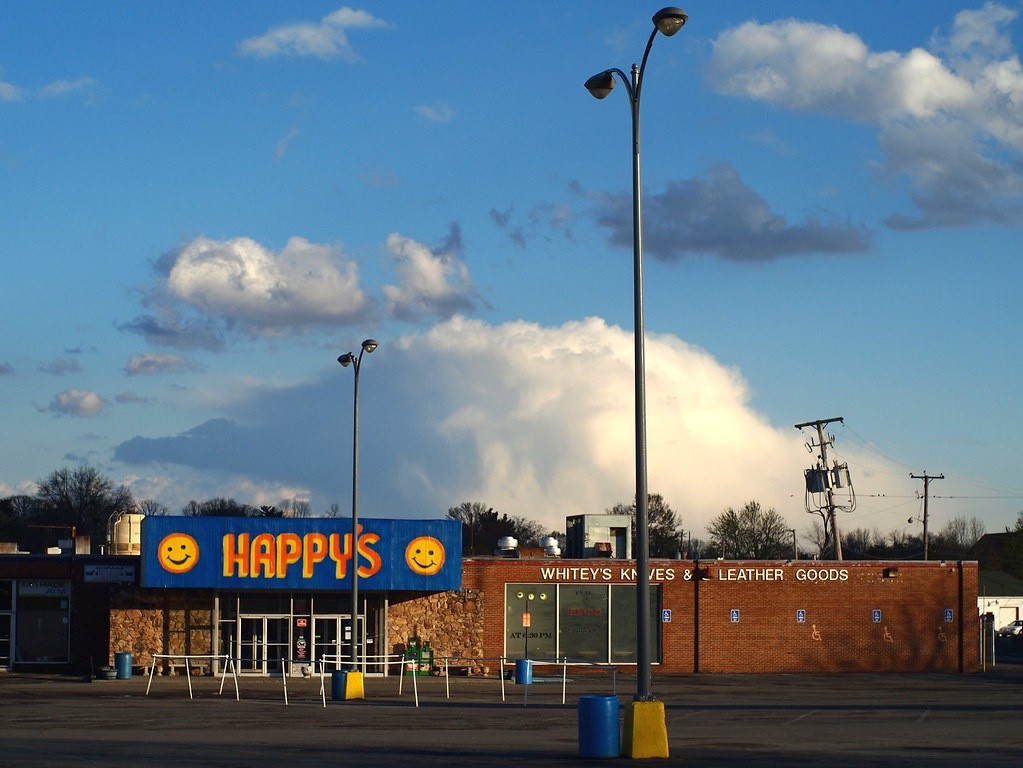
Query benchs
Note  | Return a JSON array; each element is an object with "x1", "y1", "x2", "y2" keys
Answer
[
  {"x1": 168, "y1": 664, "x2": 208, "y2": 676},
  {"x1": 435, "y1": 663, "x2": 476, "y2": 675}
]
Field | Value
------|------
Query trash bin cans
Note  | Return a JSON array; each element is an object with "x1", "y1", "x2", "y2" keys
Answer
[
  {"x1": 515, "y1": 659, "x2": 533, "y2": 685},
  {"x1": 576, "y1": 693, "x2": 621, "y2": 759},
  {"x1": 115, "y1": 651, "x2": 132, "y2": 680},
  {"x1": 331, "y1": 670, "x2": 348, "y2": 701}
]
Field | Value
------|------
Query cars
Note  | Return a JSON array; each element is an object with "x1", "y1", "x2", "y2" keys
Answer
[{"x1": 1000, "y1": 619, "x2": 1023, "y2": 637}]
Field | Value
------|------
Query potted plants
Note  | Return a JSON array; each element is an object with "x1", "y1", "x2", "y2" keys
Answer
[
  {"x1": 499, "y1": 668, "x2": 513, "y2": 678},
  {"x1": 115, "y1": 651, "x2": 132, "y2": 678},
  {"x1": 301, "y1": 664, "x2": 313, "y2": 677},
  {"x1": 102, "y1": 667, "x2": 118, "y2": 680}
]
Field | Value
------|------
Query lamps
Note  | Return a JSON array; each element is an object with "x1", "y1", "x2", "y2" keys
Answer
[{"x1": 889, "y1": 565, "x2": 900, "y2": 578}]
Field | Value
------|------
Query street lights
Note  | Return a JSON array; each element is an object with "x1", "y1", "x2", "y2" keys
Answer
[
  {"x1": 339, "y1": 340, "x2": 378, "y2": 665},
  {"x1": 585, "y1": 6, "x2": 687, "y2": 691}
]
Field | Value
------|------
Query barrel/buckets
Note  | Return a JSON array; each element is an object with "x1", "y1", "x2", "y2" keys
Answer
[
  {"x1": 115, "y1": 652, "x2": 132, "y2": 679},
  {"x1": 515, "y1": 658, "x2": 532, "y2": 684},
  {"x1": 577, "y1": 693, "x2": 621, "y2": 758},
  {"x1": 332, "y1": 670, "x2": 349, "y2": 700}
]
